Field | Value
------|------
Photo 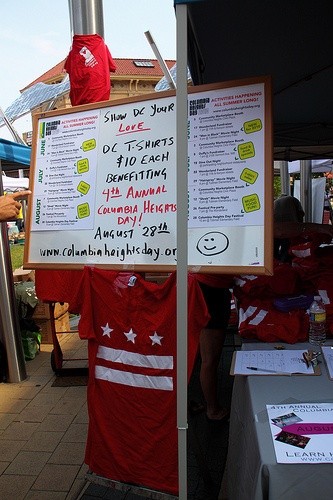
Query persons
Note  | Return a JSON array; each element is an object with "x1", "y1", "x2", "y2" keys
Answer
[
  {"x1": 273, "y1": 194, "x2": 305, "y2": 262},
  {"x1": 324, "y1": 184, "x2": 333, "y2": 211},
  {"x1": 14, "y1": 206, "x2": 23, "y2": 231},
  {"x1": 194, "y1": 222, "x2": 333, "y2": 423},
  {"x1": 0, "y1": 189, "x2": 32, "y2": 222}
]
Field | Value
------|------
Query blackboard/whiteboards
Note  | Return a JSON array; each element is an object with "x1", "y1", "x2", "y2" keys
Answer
[{"x1": 23, "y1": 75, "x2": 275, "y2": 276}]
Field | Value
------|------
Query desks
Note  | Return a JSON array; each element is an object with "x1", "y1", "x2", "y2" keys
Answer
[{"x1": 217, "y1": 339, "x2": 333, "y2": 500}]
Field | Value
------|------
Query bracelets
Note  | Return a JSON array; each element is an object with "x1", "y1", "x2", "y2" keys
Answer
[{"x1": 303, "y1": 223, "x2": 308, "y2": 232}]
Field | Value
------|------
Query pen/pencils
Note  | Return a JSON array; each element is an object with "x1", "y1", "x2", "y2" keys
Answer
[{"x1": 246, "y1": 367, "x2": 276, "y2": 372}]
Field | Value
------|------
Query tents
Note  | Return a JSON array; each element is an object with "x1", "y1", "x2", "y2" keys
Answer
[
  {"x1": 287, "y1": 157, "x2": 333, "y2": 174},
  {"x1": 171, "y1": 0, "x2": 333, "y2": 500}
]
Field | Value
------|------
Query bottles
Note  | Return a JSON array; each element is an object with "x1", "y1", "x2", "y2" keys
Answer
[{"x1": 309, "y1": 296, "x2": 326, "y2": 346}]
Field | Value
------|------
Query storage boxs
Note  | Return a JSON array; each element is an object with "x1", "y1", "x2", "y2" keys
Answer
[
  {"x1": 29, "y1": 300, "x2": 69, "y2": 320},
  {"x1": 13, "y1": 266, "x2": 35, "y2": 282},
  {"x1": 35, "y1": 312, "x2": 70, "y2": 344}
]
